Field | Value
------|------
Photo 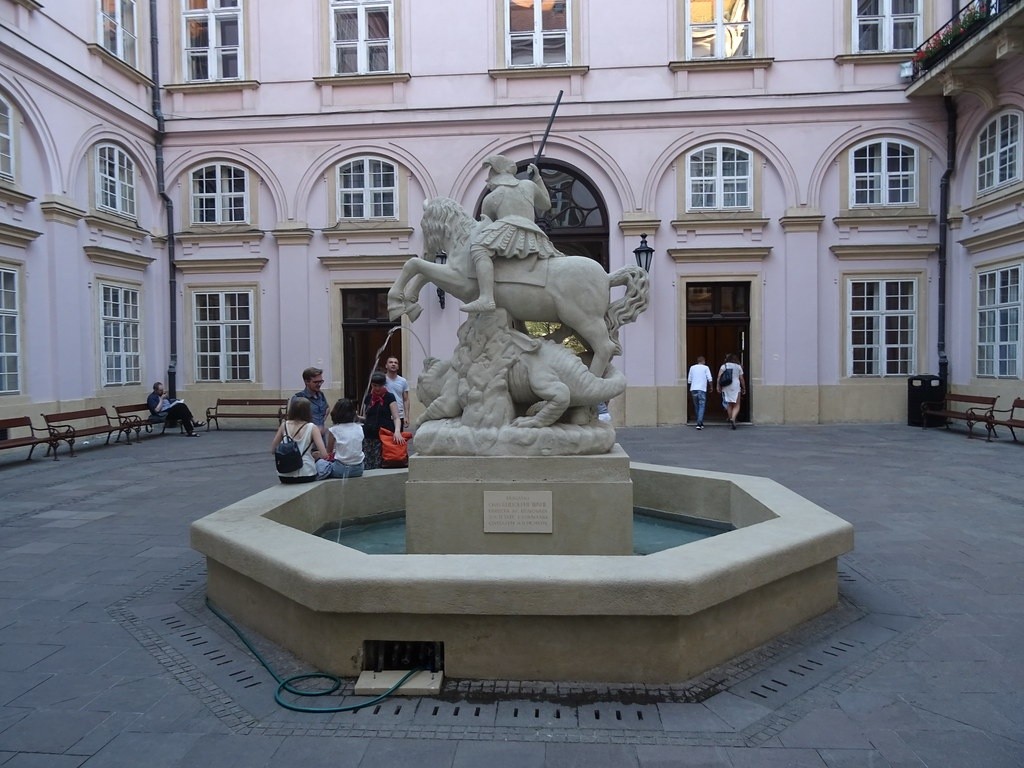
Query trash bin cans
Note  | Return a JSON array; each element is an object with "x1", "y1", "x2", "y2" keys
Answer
[{"x1": 907, "y1": 375, "x2": 947, "y2": 427}]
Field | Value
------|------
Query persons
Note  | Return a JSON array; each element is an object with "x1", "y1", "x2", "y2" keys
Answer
[
  {"x1": 382, "y1": 356, "x2": 411, "y2": 433},
  {"x1": 271, "y1": 397, "x2": 329, "y2": 485},
  {"x1": 326, "y1": 398, "x2": 365, "y2": 478},
  {"x1": 147, "y1": 382, "x2": 207, "y2": 437},
  {"x1": 361, "y1": 372, "x2": 408, "y2": 471},
  {"x1": 687, "y1": 356, "x2": 714, "y2": 430},
  {"x1": 716, "y1": 351, "x2": 747, "y2": 429},
  {"x1": 457, "y1": 155, "x2": 552, "y2": 312},
  {"x1": 289, "y1": 367, "x2": 332, "y2": 451}
]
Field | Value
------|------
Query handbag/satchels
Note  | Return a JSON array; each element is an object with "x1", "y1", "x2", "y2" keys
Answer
[
  {"x1": 378, "y1": 426, "x2": 413, "y2": 468},
  {"x1": 719, "y1": 362, "x2": 732, "y2": 387}
]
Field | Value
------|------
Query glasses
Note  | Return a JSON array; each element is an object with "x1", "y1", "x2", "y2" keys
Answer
[{"x1": 306, "y1": 380, "x2": 324, "y2": 384}]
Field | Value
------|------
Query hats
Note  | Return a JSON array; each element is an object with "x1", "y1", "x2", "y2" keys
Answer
[{"x1": 371, "y1": 373, "x2": 386, "y2": 385}]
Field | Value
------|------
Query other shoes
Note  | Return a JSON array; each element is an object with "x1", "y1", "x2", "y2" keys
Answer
[
  {"x1": 696, "y1": 423, "x2": 704, "y2": 430},
  {"x1": 727, "y1": 418, "x2": 736, "y2": 430}
]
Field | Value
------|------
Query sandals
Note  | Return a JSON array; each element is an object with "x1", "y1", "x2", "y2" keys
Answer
[
  {"x1": 193, "y1": 421, "x2": 206, "y2": 427},
  {"x1": 185, "y1": 432, "x2": 200, "y2": 437}
]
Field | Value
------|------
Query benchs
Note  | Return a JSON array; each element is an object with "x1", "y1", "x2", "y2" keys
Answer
[
  {"x1": 984, "y1": 398, "x2": 1024, "y2": 442},
  {"x1": 205, "y1": 398, "x2": 290, "y2": 433},
  {"x1": 0, "y1": 416, "x2": 61, "y2": 461},
  {"x1": 40, "y1": 407, "x2": 133, "y2": 461},
  {"x1": 112, "y1": 402, "x2": 185, "y2": 445},
  {"x1": 917, "y1": 388, "x2": 1001, "y2": 440}
]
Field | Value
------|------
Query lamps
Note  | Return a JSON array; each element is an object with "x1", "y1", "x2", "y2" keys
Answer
[
  {"x1": 632, "y1": 232, "x2": 656, "y2": 273},
  {"x1": 431, "y1": 252, "x2": 448, "y2": 310}
]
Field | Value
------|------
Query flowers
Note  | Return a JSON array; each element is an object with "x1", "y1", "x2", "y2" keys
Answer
[{"x1": 911, "y1": 7, "x2": 992, "y2": 63}]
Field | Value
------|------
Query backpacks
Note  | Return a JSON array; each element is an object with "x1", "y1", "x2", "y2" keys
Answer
[{"x1": 274, "y1": 421, "x2": 313, "y2": 474}]
